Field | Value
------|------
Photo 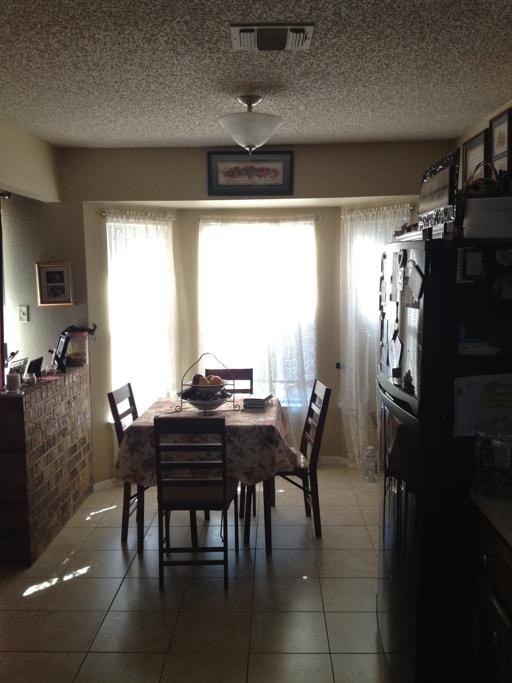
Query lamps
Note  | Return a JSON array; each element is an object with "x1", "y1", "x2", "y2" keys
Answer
[{"x1": 217, "y1": 95, "x2": 283, "y2": 151}]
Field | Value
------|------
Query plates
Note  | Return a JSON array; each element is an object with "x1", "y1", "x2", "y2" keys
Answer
[
  {"x1": 179, "y1": 390, "x2": 232, "y2": 410},
  {"x1": 188, "y1": 382, "x2": 228, "y2": 395}
]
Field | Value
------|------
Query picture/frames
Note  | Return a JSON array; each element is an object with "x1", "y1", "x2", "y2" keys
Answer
[
  {"x1": 35, "y1": 262, "x2": 74, "y2": 306},
  {"x1": 207, "y1": 151, "x2": 295, "y2": 197}
]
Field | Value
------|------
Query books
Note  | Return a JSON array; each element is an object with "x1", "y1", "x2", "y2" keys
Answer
[{"x1": 243, "y1": 392, "x2": 274, "y2": 414}]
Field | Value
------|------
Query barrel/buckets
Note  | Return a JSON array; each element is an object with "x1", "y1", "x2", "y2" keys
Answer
[{"x1": 362, "y1": 446, "x2": 380, "y2": 482}]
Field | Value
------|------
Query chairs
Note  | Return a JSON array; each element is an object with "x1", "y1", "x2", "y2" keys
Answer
[
  {"x1": 209, "y1": 368, "x2": 254, "y2": 398},
  {"x1": 108, "y1": 383, "x2": 210, "y2": 549},
  {"x1": 240, "y1": 380, "x2": 333, "y2": 547},
  {"x1": 150, "y1": 417, "x2": 239, "y2": 593}
]
